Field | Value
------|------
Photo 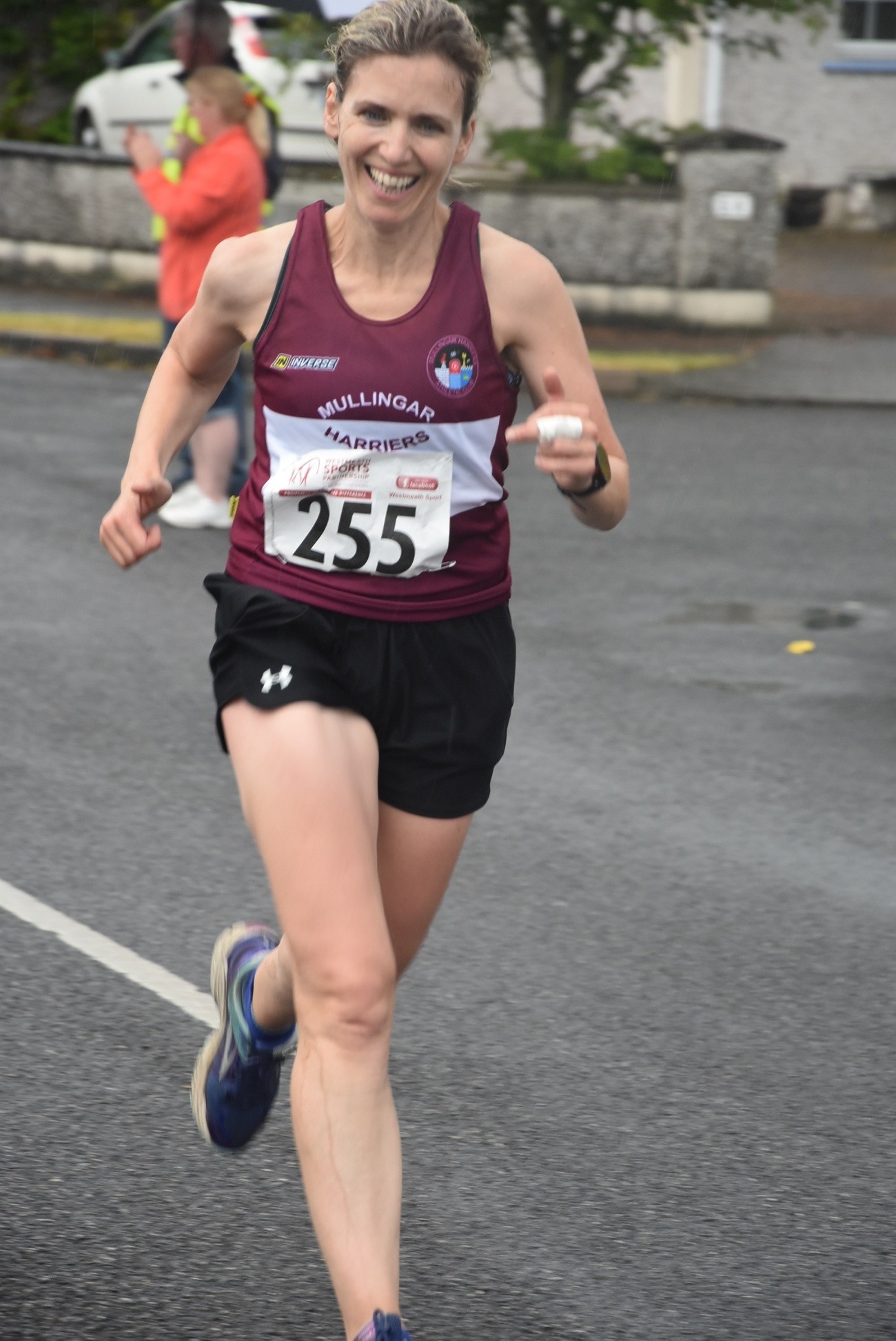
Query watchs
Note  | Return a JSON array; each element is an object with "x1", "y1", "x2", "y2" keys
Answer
[{"x1": 550, "y1": 441, "x2": 611, "y2": 497}]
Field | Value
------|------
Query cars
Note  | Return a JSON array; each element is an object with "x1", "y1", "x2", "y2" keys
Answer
[{"x1": 70, "y1": 0, "x2": 341, "y2": 167}]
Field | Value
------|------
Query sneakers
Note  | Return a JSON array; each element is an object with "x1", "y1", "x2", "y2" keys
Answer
[
  {"x1": 191, "y1": 918, "x2": 298, "y2": 1159},
  {"x1": 352, "y1": 1309, "x2": 412, "y2": 1341}
]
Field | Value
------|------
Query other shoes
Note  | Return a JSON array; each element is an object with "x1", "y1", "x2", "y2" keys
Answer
[{"x1": 157, "y1": 482, "x2": 235, "y2": 528}]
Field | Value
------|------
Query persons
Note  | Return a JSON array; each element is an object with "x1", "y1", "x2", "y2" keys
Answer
[
  {"x1": 121, "y1": 4, "x2": 280, "y2": 531},
  {"x1": 100, "y1": 0, "x2": 632, "y2": 1340}
]
